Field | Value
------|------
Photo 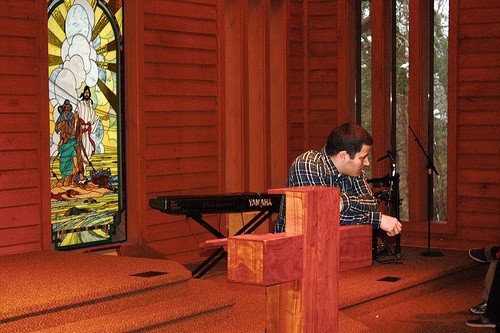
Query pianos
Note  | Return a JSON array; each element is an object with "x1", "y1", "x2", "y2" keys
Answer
[{"x1": 149, "y1": 192, "x2": 281, "y2": 216}]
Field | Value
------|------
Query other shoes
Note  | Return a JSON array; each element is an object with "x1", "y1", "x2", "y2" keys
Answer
[
  {"x1": 469, "y1": 247, "x2": 499, "y2": 263},
  {"x1": 469, "y1": 301, "x2": 488, "y2": 315},
  {"x1": 466, "y1": 318, "x2": 497, "y2": 328}
]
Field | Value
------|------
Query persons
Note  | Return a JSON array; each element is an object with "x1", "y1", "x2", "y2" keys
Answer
[
  {"x1": 465, "y1": 246, "x2": 500, "y2": 327},
  {"x1": 273, "y1": 122, "x2": 402, "y2": 236}
]
